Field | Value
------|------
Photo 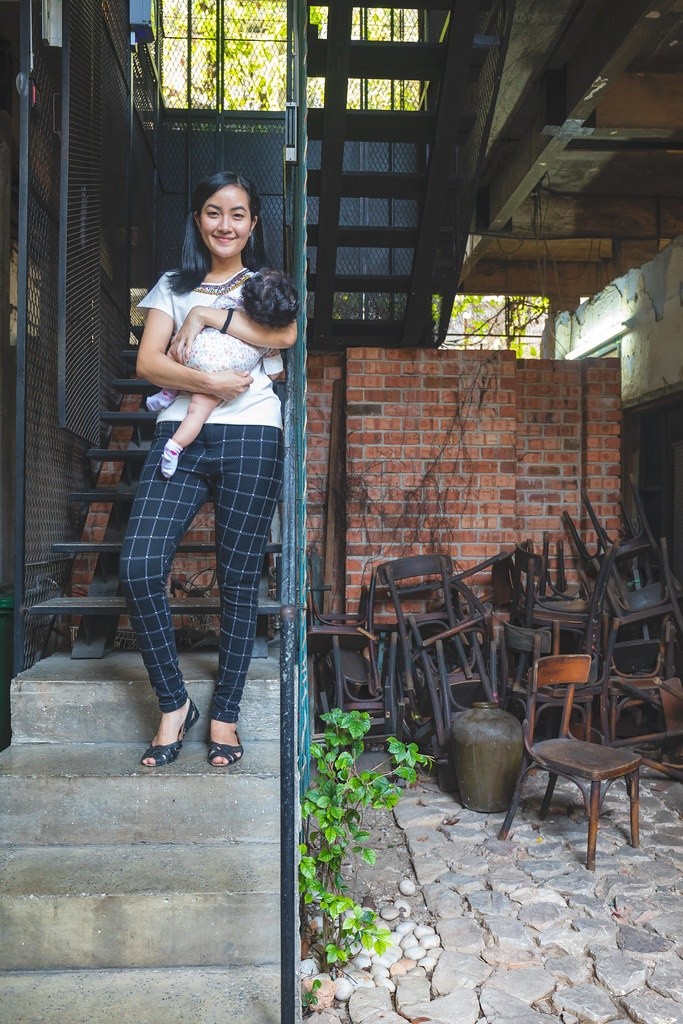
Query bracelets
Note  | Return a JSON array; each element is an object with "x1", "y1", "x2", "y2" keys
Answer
[{"x1": 220, "y1": 307, "x2": 234, "y2": 334}]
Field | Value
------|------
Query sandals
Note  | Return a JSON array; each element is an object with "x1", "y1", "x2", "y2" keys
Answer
[
  {"x1": 204, "y1": 725, "x2": 245, "y2": 770},
  {"x1": 140, "y1": 695, "x2": 199, "y2": 769}
]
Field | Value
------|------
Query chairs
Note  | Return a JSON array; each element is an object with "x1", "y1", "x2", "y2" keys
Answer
[{"x1": 306, "y1": 471, "x2": 682, "y2": 871}]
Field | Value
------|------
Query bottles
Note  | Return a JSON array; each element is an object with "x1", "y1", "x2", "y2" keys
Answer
[{"x1": 450, "y1": 699, "x2": 523, "y2": 813}]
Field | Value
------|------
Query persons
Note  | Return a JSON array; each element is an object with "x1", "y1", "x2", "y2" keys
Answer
[
  {"x1": 141, "y1": 268, "x2": 300, "y2": 478},
  {"x1": 117, "y1": 170, "x2": 298, "y2": 770}
]
